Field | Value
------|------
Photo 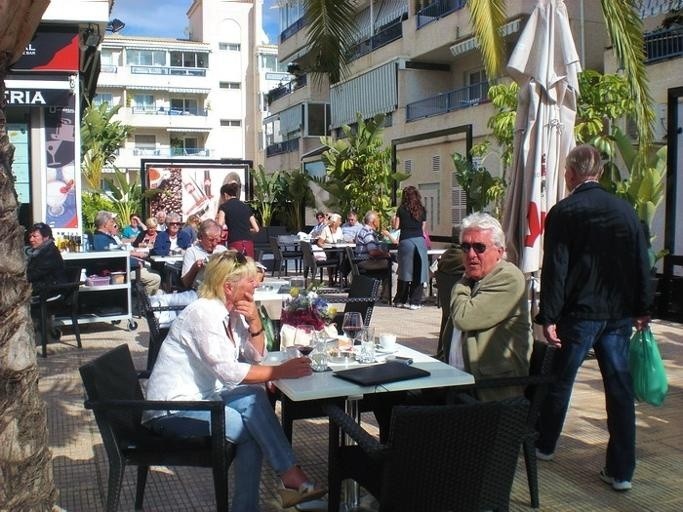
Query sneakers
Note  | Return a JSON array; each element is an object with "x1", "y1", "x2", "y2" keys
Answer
[
  {"x1": 380, "y1": 297, "x2": 423, "y2": 309},
  {"x1": 534, "y1": 448, "x2": 554, "y2": 459},
  {"x1": 599, "y1": 466, "x2": 632, "y2": 490}
]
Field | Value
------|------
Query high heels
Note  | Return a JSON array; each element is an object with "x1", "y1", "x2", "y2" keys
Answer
[{"x1": 277, "y1": 479, "x2": 325, "y2": 508}]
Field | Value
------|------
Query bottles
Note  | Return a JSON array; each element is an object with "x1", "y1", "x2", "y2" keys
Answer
[{"x1": 55, "y1": 232, "x2": 81, "y2": 253}]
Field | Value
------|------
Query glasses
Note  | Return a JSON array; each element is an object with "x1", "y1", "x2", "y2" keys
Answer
[
  {"x1": 169, "y1": 222, "x2": 181, "y2": 225},
  {"x1": 234, "y1": 247, "x2": 247, "y2": 265},
  {"x1": 461, "y1": 242, "x2": 486, "y2": 253},
  {"x1": 113, "y1": 224, "x2": 117, "y2": 229}
]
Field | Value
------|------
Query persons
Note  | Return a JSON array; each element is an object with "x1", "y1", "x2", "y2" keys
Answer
[
  {"x1": 24, "y1": 223, "x2": 68, "y2": 322},
  {"x1": 139, "y1": 250, "x2": 328, "y2": 512},
  {"x1": 91, "y1": 182, "x2": 263, "y2": 318},
  {"x1": 357, "y1": 211, "x2": 536, "y2": 511},
  {"x1": 308, "y1": 184, "x2": 434, "y2": 312},
  {"x1": 519, "y1": 144, "x2": 654, "y2": 491}
]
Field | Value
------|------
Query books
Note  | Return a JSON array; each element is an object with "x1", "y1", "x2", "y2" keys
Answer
[{"x1": 330, "y1": 361, "x2": 432, "y2": 387}]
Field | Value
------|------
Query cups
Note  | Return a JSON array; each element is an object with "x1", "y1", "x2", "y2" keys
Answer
[
  {"x1": 285, "y1": 346, "x2": 301, "y2": 361},
  {"x1": 359, "y1": 326, "x2": 376, "y2": 365},
  {"x1": 378, "y1": 333, "x2": 396, "y2": 350},
  {"x1": 310, "y1": 350, "x2": 328, "y2": 372}
]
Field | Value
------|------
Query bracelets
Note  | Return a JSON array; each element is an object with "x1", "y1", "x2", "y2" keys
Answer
[{"x1": 247, "y1": 326, "x2": 266, "y2": 336}]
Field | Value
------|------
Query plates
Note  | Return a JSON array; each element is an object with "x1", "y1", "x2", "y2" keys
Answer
[{"x1": 373, "y1": 343, "x2": 400, "y2": 354}]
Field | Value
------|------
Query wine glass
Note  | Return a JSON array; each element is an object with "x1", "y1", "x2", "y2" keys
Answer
[
  {"x1": 292, "y1": 325, "x2": 318, "y2": 371},
  {"x1": 340, "y1": 310, "x2": 365, "y2": 354}
]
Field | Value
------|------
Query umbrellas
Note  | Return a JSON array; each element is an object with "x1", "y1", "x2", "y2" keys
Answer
[{"x1": 503, "y1": 0, "x2": 585, "y2": 285}]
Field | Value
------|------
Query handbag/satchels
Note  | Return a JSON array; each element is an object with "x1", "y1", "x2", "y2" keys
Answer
[{"x1": 630, "y1": 327, "x2": 668, "y2": 406}]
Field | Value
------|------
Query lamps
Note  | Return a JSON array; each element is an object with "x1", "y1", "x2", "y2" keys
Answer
[
  {"x1": 81, "y1": 22, "x2": 102, "y2": 49},
  {"x1": 105, "y1": 17, "x2": 127, "y2": 34}
]
